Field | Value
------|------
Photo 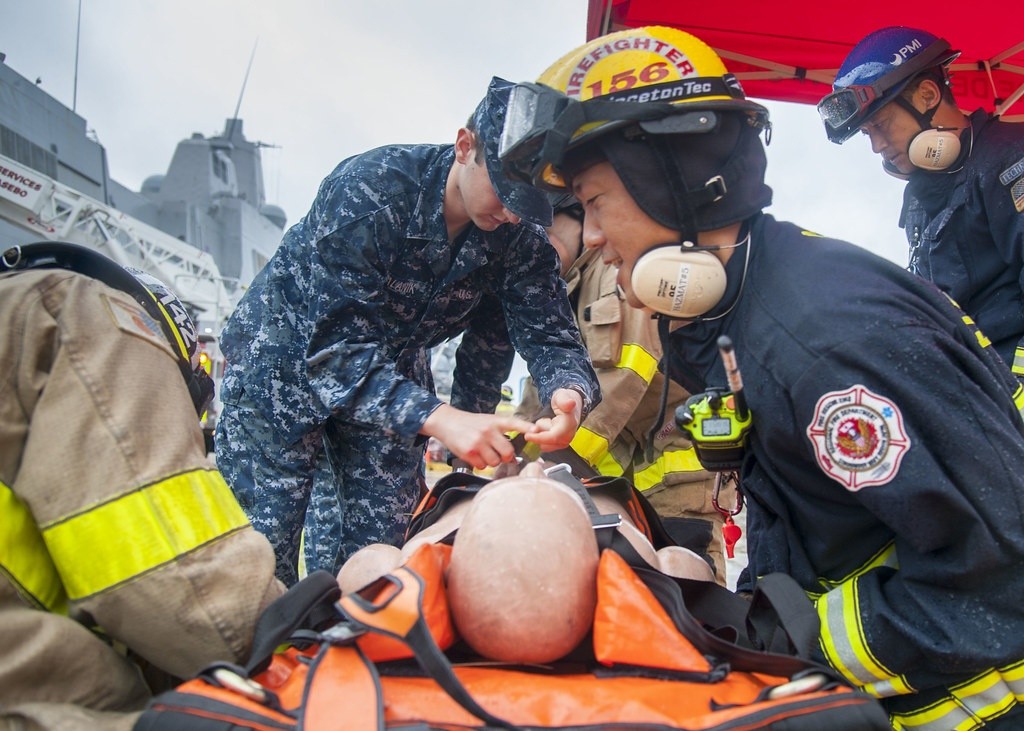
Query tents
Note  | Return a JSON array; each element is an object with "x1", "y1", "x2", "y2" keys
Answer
[{"x1": 587, "y1": 0, "x2": 1024, "y2": 124}]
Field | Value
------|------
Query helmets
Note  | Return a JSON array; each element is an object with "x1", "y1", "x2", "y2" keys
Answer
[
  {"x1": 535, "y1": 26, "x2": 769, "y2": 190},
  {"x1": 123, "y1": 265, "x2": 203, "y2": 376},
  {"x1": 825, "y1": 25, "x2": 962, "y2": 145}
]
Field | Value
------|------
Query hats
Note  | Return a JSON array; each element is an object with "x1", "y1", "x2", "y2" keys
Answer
[{"x1": 475, "y1": 77, "x2": 553, "y2": 229}]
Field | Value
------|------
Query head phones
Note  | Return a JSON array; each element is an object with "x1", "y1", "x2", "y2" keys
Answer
[
  {"x1": 882, "y1": 114, "x2": 973, "y2": 181},
  {"x1": 631, "y1": 218, "x2": 752, "y2": 320}
]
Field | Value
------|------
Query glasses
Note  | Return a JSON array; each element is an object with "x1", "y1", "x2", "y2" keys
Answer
[
  {"x1": 494, "y1": 73, "x2": 580, "y2": 174},
  {"x1": 189, "y1": 357, "x2": 216, "y2": 421},
  {"x1": 816, "y1": 81, "x2": 873, "y2": 131}
]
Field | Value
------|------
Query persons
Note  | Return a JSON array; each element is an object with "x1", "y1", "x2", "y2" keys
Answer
[
  {"x1": 817, "y1": 26, "x2": 1023, "y2": 420},
  {"x1": 406, "y1": 456, "x2": 658, "y2": 668},
  {"x1": 1, "y1": 241, "x2": 292, "y2": 731},
  {"x1": 215, "y1": 76, "x2": 602, "y2": 591},
  {"x1": 533, "y1": 25, "x2": 1024, "y2": 731}
]
